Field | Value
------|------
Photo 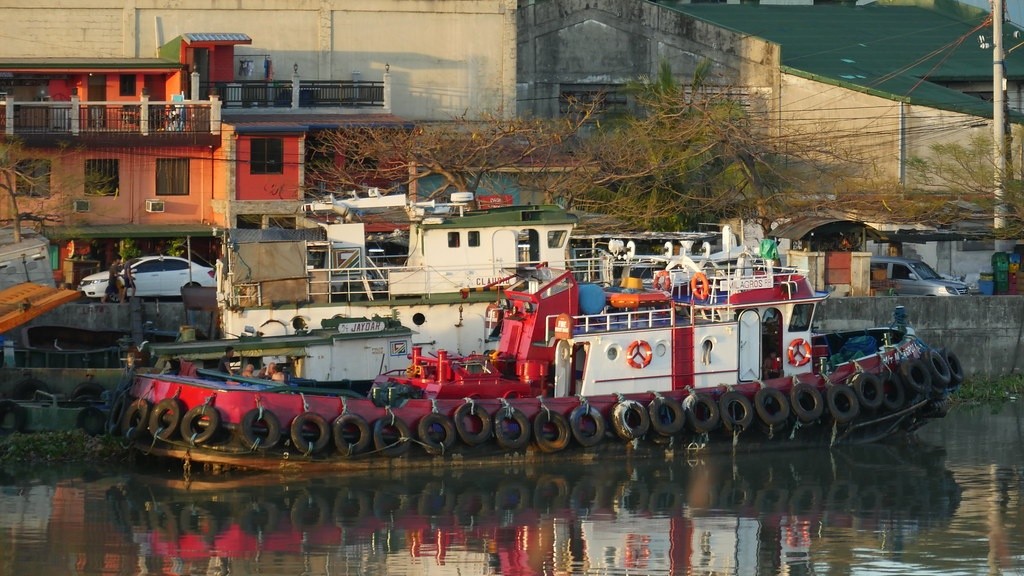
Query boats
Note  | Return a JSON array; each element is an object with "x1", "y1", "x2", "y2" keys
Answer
[
  {"x1": 108, "y1": 189, "x2": 967, "y2": 455},
  {"x1": 111, "y1": 455, "x2": 962, "y2": 575}
]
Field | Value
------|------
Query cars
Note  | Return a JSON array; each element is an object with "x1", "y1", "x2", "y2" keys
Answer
[
  {"x1": 869, "y1": 259, "x2": 966, "y2": 297},
  {"x1": 76, "y1": 255, "x2": 215, "y2": 306}
]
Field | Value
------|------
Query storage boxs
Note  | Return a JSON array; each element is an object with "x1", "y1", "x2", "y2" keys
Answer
[{"x1": 978, "y1": 250, "x2": 1021, "y2": 296}]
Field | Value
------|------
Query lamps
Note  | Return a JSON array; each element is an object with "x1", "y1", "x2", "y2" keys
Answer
[
  {"x1": 385, "y1": 62, "x2": 390, "y2": 72},
  {"x1": 293, "y1": 62, "x2": 299, "y2": 74}
]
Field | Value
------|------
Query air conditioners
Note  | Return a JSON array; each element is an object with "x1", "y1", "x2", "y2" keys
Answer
[
  {"x1": 146, "y1": 199, "x2": 165, "y2": 212},
  {"x1": 73, "y1": 199, "x2": 90, "y2": 213}
]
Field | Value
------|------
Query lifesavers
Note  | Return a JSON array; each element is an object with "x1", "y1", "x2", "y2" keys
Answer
[
  {"x1": 100, "y1": 472, "x2": 920, "y2": 541},
  {"x1": 755, "y1": 387, "x2": 791, "y2": 427},
  {"x1": 372, "y1": 414, "x2": 412, "y2": 458},
  {"x1": 653, "y1": 270, "x2": 671, "y2": 292},
  {"x1": 533, "y1": 410, "x2": 570, "y2": 451},
  {"x1": 333, "y1": 411, "x2": 370, "y2": 455},
  {"x1": 687, "y1": 395, "x2": 719, "y2": 436},
  {"x1": 825, "y1": 384, "x2": 859, "y2": 422},
  {"x1": 853, "y1": 371, "x2": 883, "y2": 410},
  {"x1": 903, "y1": 356, "x2": 931, "y2": 394},
  {"x1": 570, "y1": 404, "x2": 606, "y2": 448},
  {"x1": 107, "y1": 394, "x2": 131, "y2": 435},
  {"x1": 453, "y1": 402, "x2": 493, "y2": 447},
  {"x1": 719, "y1": 391, "x2": 755, "y2": 433},
  {"x1": 941, "y1": 349, "x2": 965, "y2": 386},
  {"x1": 788, "y1": 338, "x2": 812, "y2": 366},
  {"x1": 239, "y1": 406, "x2": 282, "y2": 450},
  {"x1": 148, "y1": 397, "x2": 184, "y2": 441},
  {"x1": 924, "y1": 350, "x2": 951, "y2": 386},
  {"x1": 625, "y1": 340, "x2": 653, "y2": 370},
  {"x1": 882, "y1": 372, "x2": 904, "y2": 410},
  {"x1": 291, "y1": 411, "x2": 331, "y2": 455},
  {"x1": 648, "y1": 395, "x2": 685, "y2": 437},
  {"x1": 611, "y1": 398, "x2": 648, "y2": 441},
  {"x1": 122, "y1": 397, "x2": 150, "y2": 441},
  {"x1": 180, "y1": 403, "x2": 219, "y2": 446},
  {"x1": 494, "y1": 406, "x2": 531, "y2": 451},
  {"x1": 417, "y1": 413, "x2": 455, "y2": 452},
  {"x1": 691, "y1": 272, "x2": 708, "y2": 300},
  {"x1": 791, "y1": 384, "x2": 826, "y2": 424}
]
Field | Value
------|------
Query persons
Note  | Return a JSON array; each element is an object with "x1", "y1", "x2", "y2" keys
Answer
[
  {"x1": 218, "y1": 346, "x2": 234, "y2": 382},
  {"x1": 101, "y1": 254, "x2": 137, "y2": 305},
  {"x1": 236, "y1": 361, "x2": 289, "y2": 381}
]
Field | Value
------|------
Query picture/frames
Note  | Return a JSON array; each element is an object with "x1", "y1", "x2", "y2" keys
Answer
[{"x1": 330, "y1": 247, "x2": 362, "y2": 276}]
